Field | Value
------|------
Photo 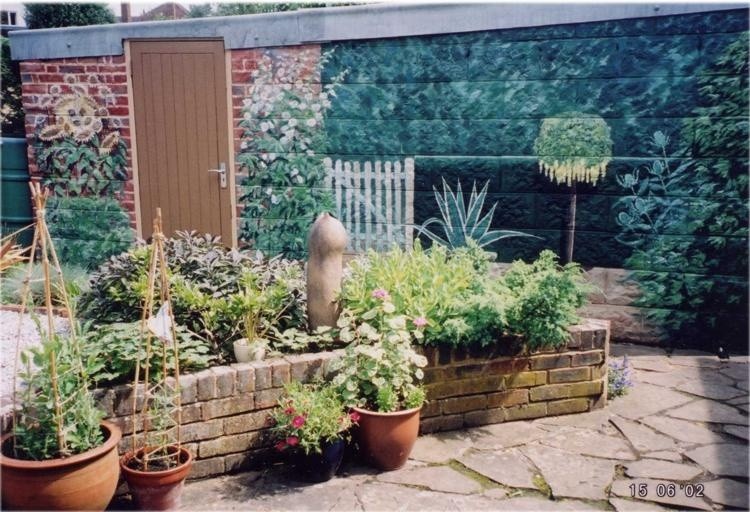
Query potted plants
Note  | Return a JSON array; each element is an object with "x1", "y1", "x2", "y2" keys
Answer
[
  {"x1": 117, "y1": 202, "x2": 197, "y2": 510},
  {"x1": 0, "y1": 179, "x2": 126, "y2": 510},
  {"x1": 217, "y1": 267, "x2": 293, "y2": 365}
]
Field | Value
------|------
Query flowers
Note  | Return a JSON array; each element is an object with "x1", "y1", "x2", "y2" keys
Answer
[
  {"x1": 263, "y1": 376, "x2": 354, "y2": 457},
  {"x1": 328, "y1": 288, "x2": 429, "y2": 414}
]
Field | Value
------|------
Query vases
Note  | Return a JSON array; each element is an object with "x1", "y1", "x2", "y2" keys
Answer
[
  {"x1": 346, "y1": 401, "x2": 419, "y2": 471},
  {"x1": 284, "y1": 441, "x2": 347, "y2": 486}
]
